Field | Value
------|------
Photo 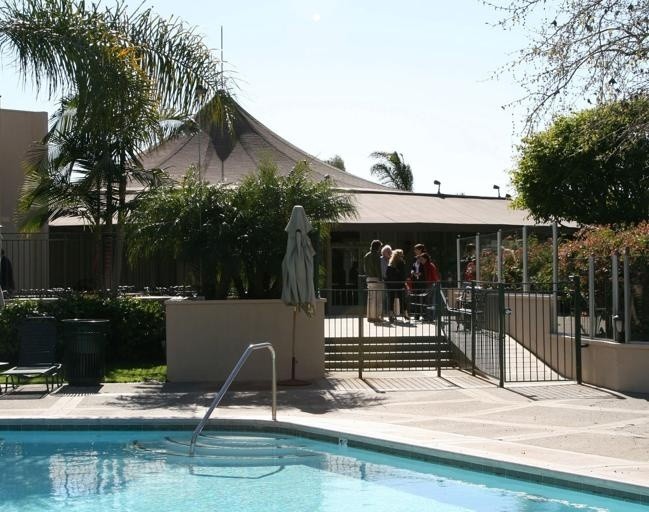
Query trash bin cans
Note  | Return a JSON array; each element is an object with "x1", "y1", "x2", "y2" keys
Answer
[{"x1": 60, "y1": 318, "x2": 111, "y2": 387}]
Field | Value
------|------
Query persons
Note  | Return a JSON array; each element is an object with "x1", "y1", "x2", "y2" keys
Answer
[
  {"x1": 414, "y1": 252, "x2": 441, "y2": 323},
  {"x1": 0, "y1": 248, "x2": 17, "y2": 299},
  {"x1": 410, "y1": 243, "x2": 427, "y2": 320},
  {"x1": 383, "y1": 248, "x2": 411, "y2": 323},
  {"x1": 405, "y1": 269, "x2": 418, "y2": 312},
  {"x1": 380, "y1": 245, "x2": 393, "y2": 314},
  {"x1": 461, "y1": 234, "x2": 524, "y2": 292},
  {"x1": 363, "y1": 239, "x2": 387, "y2": 324}
]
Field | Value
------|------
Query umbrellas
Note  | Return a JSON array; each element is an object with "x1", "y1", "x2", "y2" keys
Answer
[{"x1": 280, "y1": 204, "x2": 319, "y2": 379}]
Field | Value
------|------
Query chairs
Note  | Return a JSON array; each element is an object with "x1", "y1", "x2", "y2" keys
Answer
[
  {"x1": 431, "y1": 281, "x2": 484, "y2": 334},
  {"x1": 408, "y1": 283, "x2": 436, "y2": 321},
  {"x1": 0, "y1": 316, "x2": 63, "y2": 394}
]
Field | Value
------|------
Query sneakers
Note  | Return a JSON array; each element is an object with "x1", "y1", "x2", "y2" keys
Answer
[{"x1": 367, "y1": 314, "x2": 434, "y2": 325}]
